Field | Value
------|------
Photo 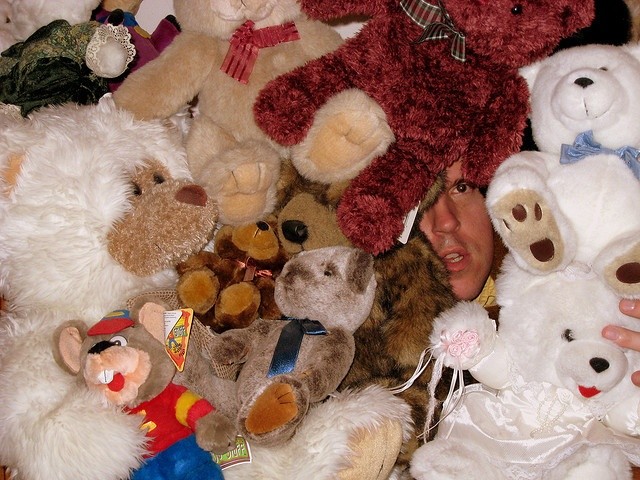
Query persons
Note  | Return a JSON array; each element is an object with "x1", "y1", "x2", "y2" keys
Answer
[{"x1": 419, "y1": 154, "x2": 640, "y2": 390}]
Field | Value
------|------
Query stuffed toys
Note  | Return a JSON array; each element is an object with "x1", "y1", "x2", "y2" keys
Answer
[
  {"x1": 275, "y1": 157, "x2": 480, "y2": 480},
  {"x1": 0, "y1": 0, "x2": 136, "y2": 112},
  {"x1": 409, "y1": 250, "x2": 640, "y2": 480},
  {"x1": 252, "y1": 0, "x2": 597, "y2": 254},
  {"x1": 0, "y1": 102, "x2": 417, "y2": 480},
  {"x1": 182, "y1": 246, "x2": 378, "y2": 448},
  {"x1": 486, "y1": 38, "x2": 640, "y2": 299},
  {"x1": 49, "y1": 293, "x2": 236, "y2": 480},
  {"x1": 92, "y1": 0, "x2": 182, "y2": 94},
  {"x1": 173, "y1": 213, "x2": 282, "y2": 332},
  {"x1": 114, "y1": 0, "x2": 397, "y2": 228}
]
